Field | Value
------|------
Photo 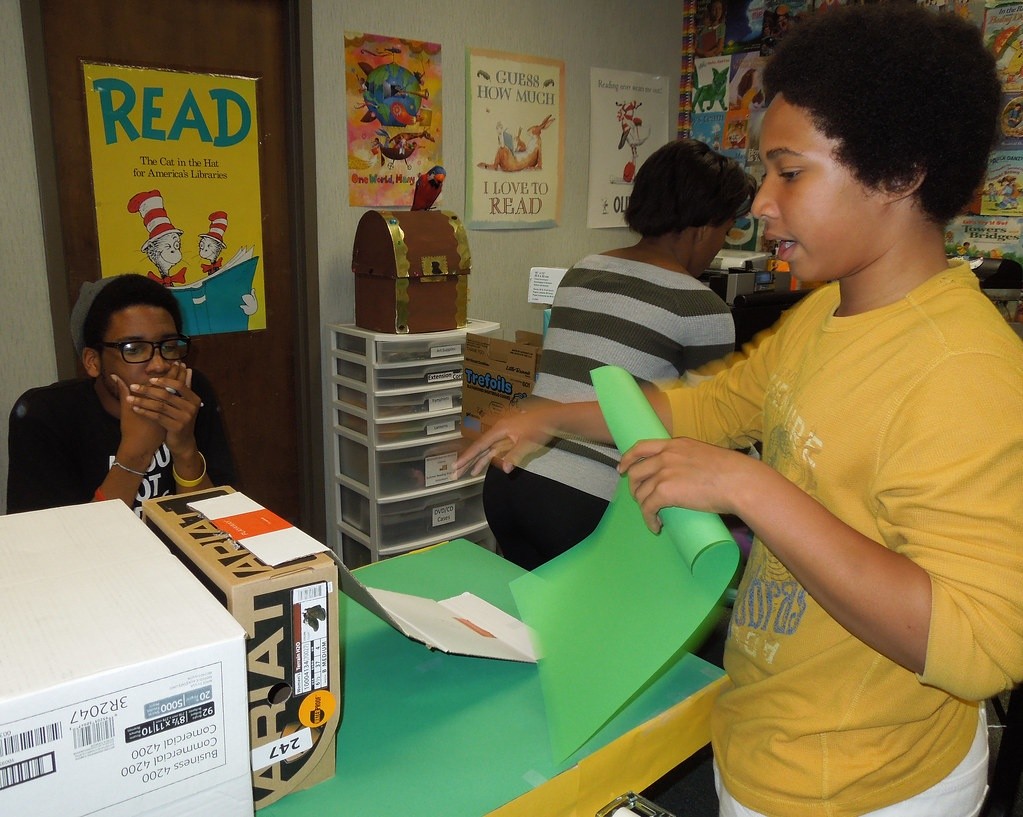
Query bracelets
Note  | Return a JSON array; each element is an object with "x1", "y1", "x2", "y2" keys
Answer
[
  {"x1": 171, "y1": 451, "x2": 207, "y2": 488},
  {"x1": 95, "y1": 488, "x2": 108, "y2": 502},
  {"x1": 112, "y1": 461, "x2": 147, "y2": 476}
]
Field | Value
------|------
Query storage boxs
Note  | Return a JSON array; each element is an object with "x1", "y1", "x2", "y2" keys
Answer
[
  {"x1": 349, "y1": 208, "x2": 473, "y2": 335},
  {"x1": 136, "y1": 485, "x2": 346, "y2": 813},
  {"x1": 458, "y1": 327, "x2": 545, "y2": 444},
  {"x1": 327, "y1": 315, "x2": 505, "y2": 570},
  {"x1": 0, "y1": 496, "x2": 264, "y2": 813}
]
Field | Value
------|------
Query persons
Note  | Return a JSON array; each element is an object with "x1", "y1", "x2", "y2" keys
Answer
[
  {"x1": 439, "y1": 1, "x2": 1023, "y2": 817},
  {"x1": 481, "y1": 138, "x2": 761, "y2": 573},
  {"x1": 7, "y1": 271, "x2": 239, "y2": 524}
]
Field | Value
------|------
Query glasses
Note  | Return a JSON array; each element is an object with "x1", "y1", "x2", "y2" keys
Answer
[{"x1": 103, "y1": 334, "x2": 191, "y2": 364}]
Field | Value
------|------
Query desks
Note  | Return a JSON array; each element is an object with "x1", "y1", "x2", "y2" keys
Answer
[{"x1": 248, "y1": 536, "x2": 729, "y2": 817}]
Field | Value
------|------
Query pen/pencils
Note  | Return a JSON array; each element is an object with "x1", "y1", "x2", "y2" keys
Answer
[{"x1": 164, "y1": 386, "x2": 204, "y2": 407}]
